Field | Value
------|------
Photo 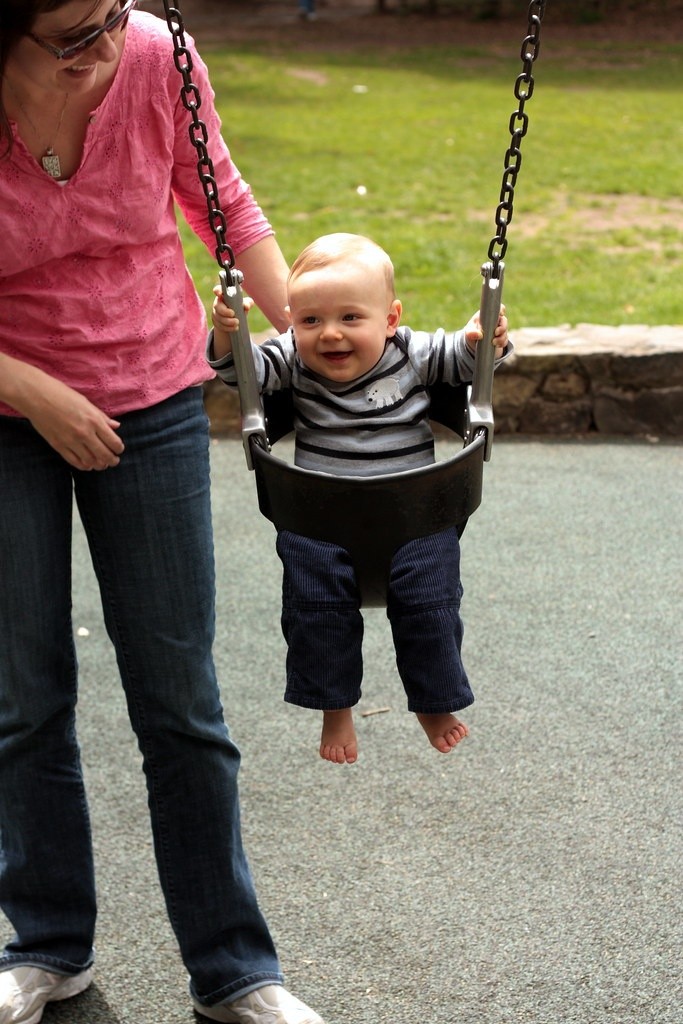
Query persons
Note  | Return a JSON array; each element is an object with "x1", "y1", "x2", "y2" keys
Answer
[
  {"x1": 206, "y1": 233, "x2": 515, "y2": 765},
  {"x1": 0, "y1": 0, "x2": 322, "y2": 1024}
]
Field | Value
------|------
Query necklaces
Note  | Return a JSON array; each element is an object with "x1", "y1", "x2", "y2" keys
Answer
[{"x1": 7, "y1": 78, "x2": 69, "y2": 178}]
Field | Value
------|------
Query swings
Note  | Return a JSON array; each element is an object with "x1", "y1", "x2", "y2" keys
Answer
[{"x1": 158, "y1": 0, "x2": 549, "y2": 610}]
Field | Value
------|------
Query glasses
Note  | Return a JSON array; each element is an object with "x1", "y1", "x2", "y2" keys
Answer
[{"x1": 0, "y1": 0, "x2": 138, "y2": 70}]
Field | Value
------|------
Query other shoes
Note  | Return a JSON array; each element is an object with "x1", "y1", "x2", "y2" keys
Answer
[
  {"x1": 193, "y1": 985, "x2": 324, "y2": 1024},
  {"x1": 0, "y1": 963, "x2": 92, "y2": 1024}
]
